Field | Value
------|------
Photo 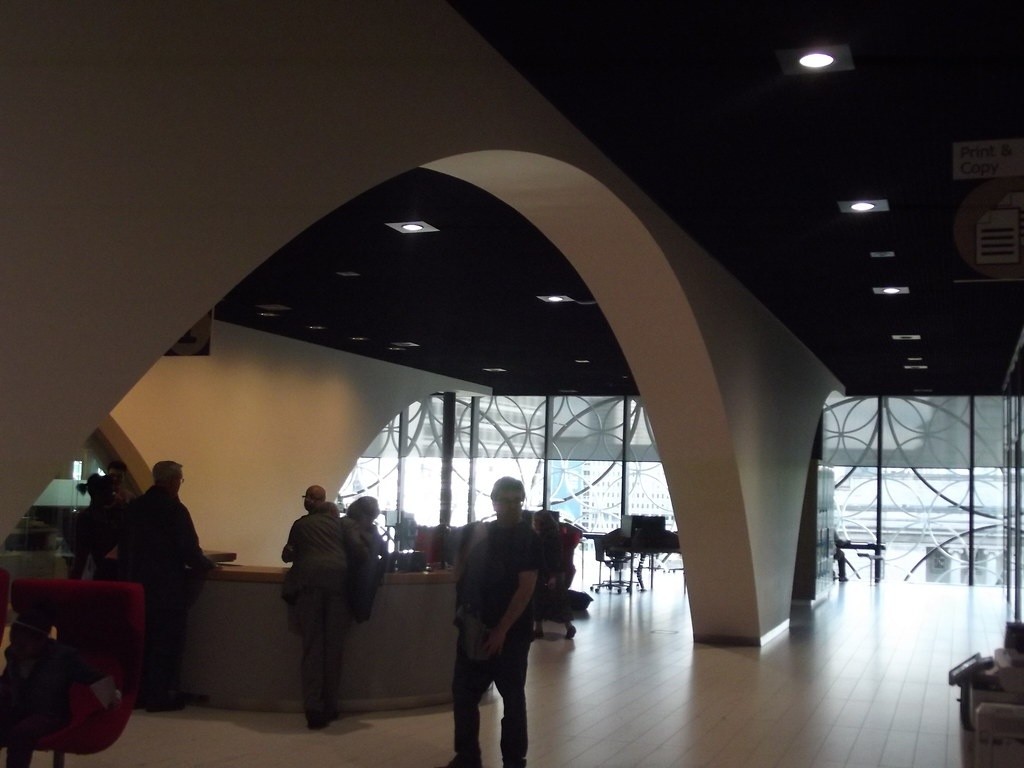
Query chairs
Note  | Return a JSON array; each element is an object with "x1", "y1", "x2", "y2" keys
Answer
[{"x1": 7, "y1": 580, "x2": 143, "y2": 767}]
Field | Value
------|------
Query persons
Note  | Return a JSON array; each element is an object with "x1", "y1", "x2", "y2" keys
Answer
[
  {"x1": 834, "y1": 529, "x2": 851, "y2": 582},
  {"x1": 292, "y1": 485, "x2": 326, "y2": 527},
  {"x1": 346, "y1": 496, "x2": 389, "y2": 564},
  {"x1": 281, "y1": 502, "x2": 369, "y2": 730},
  {"x1": 118, "y1": 461, "x2": 224, "y2": 713},
  {"x1": 532, "y1": 509, "x2": 576, "y2": 640},
  {"x1": 0, "y1": 611, "x2": 123, "y2": 768},
  {"x1": 67, "y1": 461, "x2": 137, "y2": 581},
  {"x1": 446, "y1": 477, "x2": 543, "y2": 768}
]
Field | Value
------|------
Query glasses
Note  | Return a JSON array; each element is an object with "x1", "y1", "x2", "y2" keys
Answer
[
  {"x1": 175, "y1": 479, "x2": 184, "y2": 484},
  {"x1": 495, "y1": 498, "x2": 521, "y2": 504},
  {"x1": 302, "y1": 496, "x2": 306, "y2": 499}
]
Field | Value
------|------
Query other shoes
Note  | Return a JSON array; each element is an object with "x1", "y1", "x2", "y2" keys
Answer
[
  {"x1": 306, "y1": 708, "x2": 338, "y2": 729},
  {"x1": 137, "y1": 691, "x2": 184, "y2": 711},
  {"x1": 435, "y1": 754, "x2": 481, "y2": 768},
  {"x1": 502, "y1": 758, "x2": 527, "y2": 768},
  {"x1": 565, "y1": 626, "x2": 576, "y2": 639},
  {"x1": 533, "y1": 630, "x2": 544, "y2": 637}
]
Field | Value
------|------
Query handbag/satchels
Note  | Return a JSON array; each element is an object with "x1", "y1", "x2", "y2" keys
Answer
[{"x1": 462, "y1": 612, "x2": 489, "y2": 661}]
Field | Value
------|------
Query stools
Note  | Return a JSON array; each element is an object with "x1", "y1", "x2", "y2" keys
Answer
[
  {"x1": 858, "y1": 553, "x2": 884, "y2": 586},
  {"x1": 590, "y1": 555, "x2": 636, "y2": 594},
  {"x1": 977, "y1": 704, "x2": 1023, "y2": 768}
]
